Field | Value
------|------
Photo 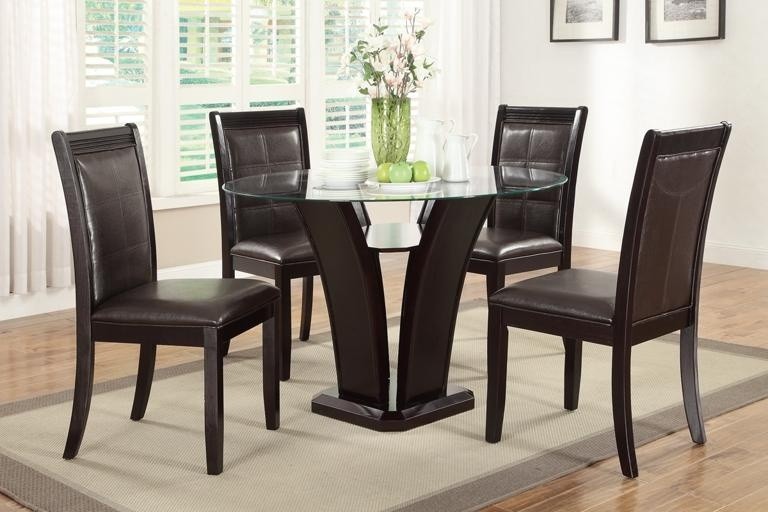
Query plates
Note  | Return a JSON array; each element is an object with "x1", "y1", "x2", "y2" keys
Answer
[
  {"x1": 313, "y1": 187, "x2": 375, "y2": 201},
  {"x1": 366, "y1": 176, "x2": 440, "y2": 190},
  {"x1": 319, "y1": 150, "x2": 371, "y2": 185}
]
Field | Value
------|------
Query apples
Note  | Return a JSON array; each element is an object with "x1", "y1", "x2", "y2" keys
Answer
[{"x1": 377, "y1": 160, "x2": 431, "y2": 183}]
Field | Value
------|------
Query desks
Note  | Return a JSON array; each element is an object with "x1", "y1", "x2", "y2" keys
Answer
[{"x1": 222, "y1": 160, "x2": 570, "y2": 432}]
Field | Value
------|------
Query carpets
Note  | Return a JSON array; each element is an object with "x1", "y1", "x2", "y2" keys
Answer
[{"x1": 0, "y1": 298, "x2": 768, "y2": 512}]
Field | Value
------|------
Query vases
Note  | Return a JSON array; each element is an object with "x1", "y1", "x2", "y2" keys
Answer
[{"x1": 372, "y1": 96, "x2": 411, "y2": 167}]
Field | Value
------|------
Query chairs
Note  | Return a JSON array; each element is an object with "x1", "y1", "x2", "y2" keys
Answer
[
  {"x1": 486, "y1": 121, "x2": 730, "y2": 478},
  {"x1": 50, "y1": 123, "x2": 283, "y2": 475},
  {"x1": 210, "y1": 106, "x2": 320, "y2": 381},
  {"x1": 466, "y1": 104, "x2": 588, "y2": 310}
]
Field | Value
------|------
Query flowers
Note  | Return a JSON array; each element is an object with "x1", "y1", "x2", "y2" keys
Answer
[{"x1": 336, "y1": 8, "x2": 443, "y2": 155}]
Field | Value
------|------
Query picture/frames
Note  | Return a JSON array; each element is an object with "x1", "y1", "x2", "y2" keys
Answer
[
  {"x1": 550, "y1": 0, "x2": 620, "y2": 42},
  {"x1": 645, "y1": 0, "x2": 726, "y2": 43}
]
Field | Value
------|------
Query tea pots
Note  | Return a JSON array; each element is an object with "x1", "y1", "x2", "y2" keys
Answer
[
  {"x1": 412, "y1": 119, "x2": 454, "y2": 179},
  {"x1": 442, "y1": 132, "x2": 479, "y2": 182}
]
